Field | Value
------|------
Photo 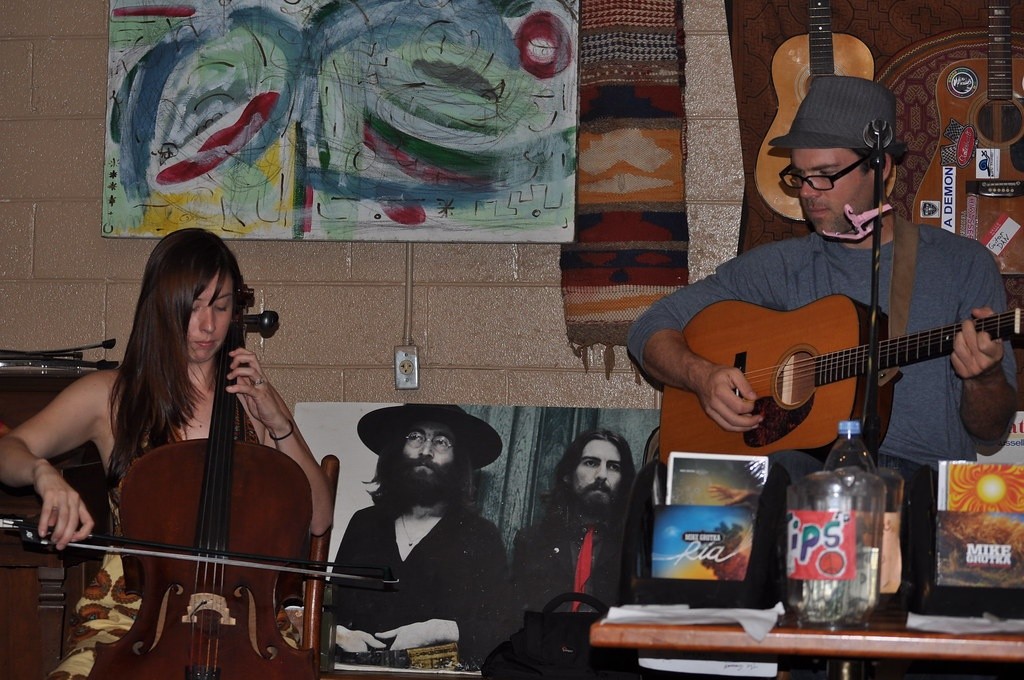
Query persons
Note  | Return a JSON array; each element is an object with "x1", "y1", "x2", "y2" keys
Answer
[
  {"x1": 0, "y1": 228, "x2": 332, "y2": 680},
  {"x1": 329, "y1": 404, "x2": 635, "y2": 672},
  {"x1": 627, "y1": 76, "x2": 1017, "y2": 587}
]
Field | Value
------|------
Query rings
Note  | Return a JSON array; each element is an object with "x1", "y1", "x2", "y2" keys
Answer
[{"x1": 252, "y1": 376, "x2": 265, "y2": 386}]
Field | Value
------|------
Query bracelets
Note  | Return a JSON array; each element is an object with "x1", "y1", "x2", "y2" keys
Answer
[{"x1": 269, "y1": 419, "x2": 294, "y2": 440}]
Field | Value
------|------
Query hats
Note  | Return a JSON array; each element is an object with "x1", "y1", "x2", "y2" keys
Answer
[
  {"x1": 357, "y1": 403, "x2": 502, "y2": 469},
  {"x1": 768, "y1": 75, "x2": 909, "y2": 155}
]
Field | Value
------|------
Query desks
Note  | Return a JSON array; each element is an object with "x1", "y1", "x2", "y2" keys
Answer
[
  {"x1": 589, "y1": 613, "x2": 1024, "y2": 680},
  {"x1": 0, "y1": 496, "x2": 112, "y2": 680}
]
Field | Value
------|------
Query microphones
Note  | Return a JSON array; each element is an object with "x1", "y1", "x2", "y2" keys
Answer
[{"x1": 863, "y1": 118, "x2": 893, "y2": 149}]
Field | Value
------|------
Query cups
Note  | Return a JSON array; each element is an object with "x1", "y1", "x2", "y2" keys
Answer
[{"x1": 785, "y1": 463, "x2": 904, "y2": 630}]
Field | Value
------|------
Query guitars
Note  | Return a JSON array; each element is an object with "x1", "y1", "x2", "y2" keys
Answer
[
  {"x1": 653, "y1": 290, "x2": 1022, "y2": 475},
  {"x1": 753, "y1": 0, "x2": 898, "y2": 226},
  {"x1": 908, "y1": 1, "x2": 1024, "y2": 275}
]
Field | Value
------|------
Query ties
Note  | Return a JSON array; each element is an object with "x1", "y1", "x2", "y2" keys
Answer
[{"x1": 573, "y1": 524, "x2": 596, "y2": 613}]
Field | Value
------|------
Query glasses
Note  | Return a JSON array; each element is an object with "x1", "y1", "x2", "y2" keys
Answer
[
  {"x1": 779, "y1": 152, "x2": 872, "y2": 190},
  {"x1": 403, "y1": 431, "x2": 454, "y2": 451}
]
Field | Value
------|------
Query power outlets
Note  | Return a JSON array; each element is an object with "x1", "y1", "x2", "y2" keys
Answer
[{"x1": 394, "y1": 346, "x2": 419, "y2": 390}]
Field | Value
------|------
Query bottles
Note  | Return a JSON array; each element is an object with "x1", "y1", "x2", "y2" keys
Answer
[{"x1": 822, "y1": 420, "x2": 877, "y2": 477}]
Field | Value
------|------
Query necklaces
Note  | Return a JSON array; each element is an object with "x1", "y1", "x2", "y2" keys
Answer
[{"x1": 402, "y1": 512, "x2": 427, "y2": 545}]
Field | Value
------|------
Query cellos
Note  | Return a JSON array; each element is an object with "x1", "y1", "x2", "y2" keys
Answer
[{"x1": 91, "y1": 265, "x2": 321, "y2": 680}]
